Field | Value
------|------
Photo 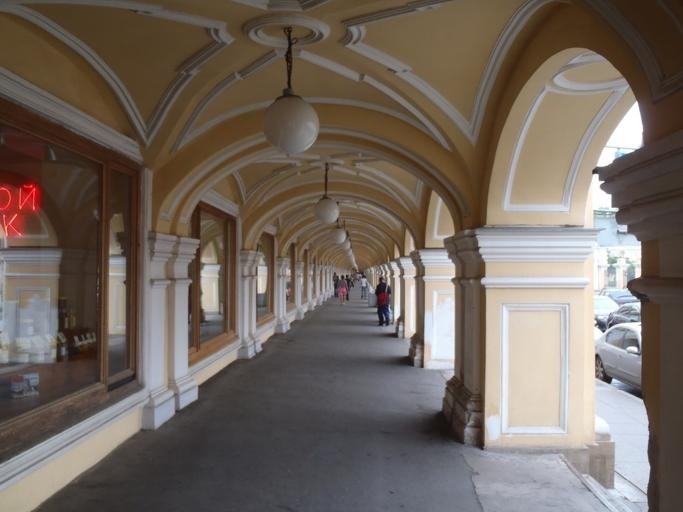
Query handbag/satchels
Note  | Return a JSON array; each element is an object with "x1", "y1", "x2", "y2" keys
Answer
[{"x1": 378, "y1": 293, "x2": 389, "y2": 305}]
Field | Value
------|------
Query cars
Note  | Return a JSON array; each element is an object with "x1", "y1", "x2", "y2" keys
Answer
[{"x1": 592, "y1": 288, "x2": 641, "y2": 392}]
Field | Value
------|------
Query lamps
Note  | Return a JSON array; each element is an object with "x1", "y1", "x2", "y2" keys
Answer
[
  {"x1": 264, "y1": 25, "x2": 319, "y2": 153},
  {"x1": 312, "y1": 162, "x2": 353, "y2": 256}
]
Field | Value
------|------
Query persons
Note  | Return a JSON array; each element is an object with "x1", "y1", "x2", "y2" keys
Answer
[
  {"x1": 344, "y1": 275, "x2": 354, "y2": 301},
  {"x1": 359, "y1": 275, "x2": 368, "y2": 299},
  {"x1": 349, "y1": 271, "x2": 364, "y2": 288},
  {"x1": 332, "y1": 272, "x2": 339, "y2": 298},
  {"x1": 336, "y1": 274, "x2": 348, "y2": 305},
  {"x1": 594, "y1": 312, "x2": 617, "y2": 333},
  {"x1": 374, "y1": 276, "x2": 391, "y2": 327}
]
